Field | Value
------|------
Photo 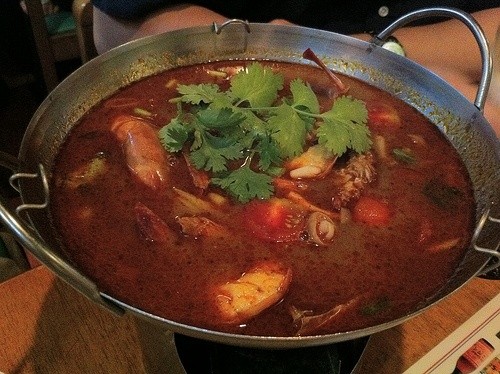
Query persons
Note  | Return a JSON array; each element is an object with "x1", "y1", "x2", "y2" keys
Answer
[{"x1": 87, "y1": 0, "x2": 500, "y2": 139}]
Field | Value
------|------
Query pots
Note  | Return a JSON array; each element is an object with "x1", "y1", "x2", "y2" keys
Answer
[{"x1": 0, "y1": 7, "x2": 500, "y2": 347}]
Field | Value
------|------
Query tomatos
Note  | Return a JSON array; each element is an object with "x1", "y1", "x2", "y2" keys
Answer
[{"x1": 241, "y1": 198, "x2": 306, "y2": 243}]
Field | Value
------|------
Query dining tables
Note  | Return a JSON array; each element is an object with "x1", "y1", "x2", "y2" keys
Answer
[{"x1": 0, "y1": 264, "x2": 499, "y2": 374}]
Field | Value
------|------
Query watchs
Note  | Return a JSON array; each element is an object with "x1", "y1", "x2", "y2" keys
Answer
[{"x1": 370, "y1": 29, "x2": 407, "y2": 60}]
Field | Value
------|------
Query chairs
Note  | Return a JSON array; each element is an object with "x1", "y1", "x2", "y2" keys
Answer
[{"x1": 24, "y1": 0, "x2": 82, "y2": 94}]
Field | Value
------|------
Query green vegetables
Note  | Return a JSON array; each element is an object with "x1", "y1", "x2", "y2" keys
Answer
[{"x1": 158, "y1": 61, "x2": 373, "y2": 203}]
